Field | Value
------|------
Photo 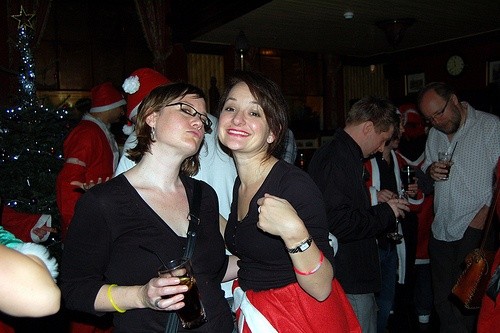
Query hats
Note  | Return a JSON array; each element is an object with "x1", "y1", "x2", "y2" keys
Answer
[
  {"x1": 90, "y1": 82, "x2": 127, "y2": 113},
  {"x1": 399, "y1": 103, "x2": 425, "y2": 137},
  {"x1": 122, "y1": 68, "x2": 174, "y2": 121}
]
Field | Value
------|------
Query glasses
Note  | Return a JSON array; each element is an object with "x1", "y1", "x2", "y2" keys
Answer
[
  {"x1": 424, "y1": 97, "x2": 451, "y2": 124},
  {"x1": 164, "y1": 102, "x2": 213, "y2": 126}
]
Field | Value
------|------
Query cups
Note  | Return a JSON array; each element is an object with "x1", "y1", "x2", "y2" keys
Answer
[
  {"x1": 157, "y1": 258, "x2": 208, "y2": 330},
  {"x1": 438, "y1": 152, "x2": 452, "y2": 180},
  {"x1": 400, "y1": 166, "x2": 416, "y2": 191}
]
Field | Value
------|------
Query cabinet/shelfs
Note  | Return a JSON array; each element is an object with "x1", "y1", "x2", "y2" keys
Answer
[
  {"x1": 225, "y1": 50, "x2": 322, "y2": 95},
  {"x1": 33, "y1": 40, "x2": 107, "y2": 91}
]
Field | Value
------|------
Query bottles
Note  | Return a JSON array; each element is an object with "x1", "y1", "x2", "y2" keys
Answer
[{"x1": 299, "y1": 154, "x2": 305, "y2": 168}]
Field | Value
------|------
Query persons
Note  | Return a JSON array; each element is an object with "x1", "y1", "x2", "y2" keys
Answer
[
  {"x1": 0, "y1": 204, "x2": 56, "y2": 244},
  {"x1": 308, "y1": 95, "x2": 424, "y2": 332},
  {"x1": 218, "y1": 72, "x2": 362, "y2": 332},
  {"x1": 283, "y1": 127, "x2": 298, "y2": 164},
  {"x1": 70, "y1": 67, "x2": 237, "y2": 220},
  {"x1": 418, "y1": 88, "x2": 500, "y2": 333},
  {"x1": 55, "y1": 84, "x2": 129, "y2": 238},
  {"x1": 61, "y1": 83, "x2": 240, "y2": 332},
  {"x1": 0, "y1": 226, "x2": 60, "y2": 318}
]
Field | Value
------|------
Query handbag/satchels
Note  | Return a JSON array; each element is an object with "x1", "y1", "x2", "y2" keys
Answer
[{"x1": 447, "y1": 249, "x2": 491, "y2": 317}]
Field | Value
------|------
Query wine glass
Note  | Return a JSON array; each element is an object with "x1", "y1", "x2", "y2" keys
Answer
[{"x1": 387, "y1": 192, "x2": 409, "y2": 239}]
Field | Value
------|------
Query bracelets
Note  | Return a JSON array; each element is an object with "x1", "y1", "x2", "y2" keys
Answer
[
  {"x1": 293, "y1": 250, "x2": 323, "y2": 276},
  {"x1": 107, "y1": 284, "x2": 127, "y2": 312}
]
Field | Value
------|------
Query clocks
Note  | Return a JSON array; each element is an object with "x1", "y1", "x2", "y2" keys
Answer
[{"x1": 447, "y1": 56, "x2": 464, "y2": 76}]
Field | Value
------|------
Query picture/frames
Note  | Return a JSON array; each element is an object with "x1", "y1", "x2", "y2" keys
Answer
[
  {"x1": 405, "y1": 71, "x2": 427, "y2": 97},
  {"x1": 486, "y1": 59, "x2": 500, "y2": 86}
]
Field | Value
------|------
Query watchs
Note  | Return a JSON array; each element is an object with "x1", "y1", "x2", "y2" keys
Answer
[{"x1": 287, "y1": 232, "x2": 312, "y2": 254}]
